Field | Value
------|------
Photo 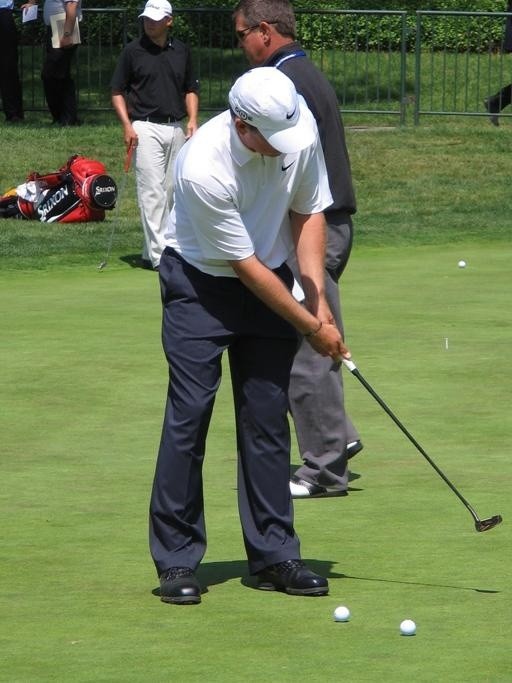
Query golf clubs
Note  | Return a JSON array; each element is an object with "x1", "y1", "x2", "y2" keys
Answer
[
  {"x1": 97, "y1": 142, "x2": 132, "y2": 270},
  {"x1": 338, "y1": 353, "x2": 502, "y2": 531}
]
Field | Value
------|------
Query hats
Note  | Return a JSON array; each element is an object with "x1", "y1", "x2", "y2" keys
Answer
[
  {"x1": 137, "y1": 0, "x2": 172, "y2": 21},
  {"x1": 229, "y1": 68, "x2": 316, "y2": 153}
]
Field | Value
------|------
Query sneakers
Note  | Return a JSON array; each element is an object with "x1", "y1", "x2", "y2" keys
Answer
[
  {"x1": 287, "y1": 471, "x2": 347, "y2": 499},
  {"x1": 346, "y1": 441, "x2": 362, "y2": 458}
]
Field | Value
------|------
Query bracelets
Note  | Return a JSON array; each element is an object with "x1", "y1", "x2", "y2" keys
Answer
[{"x1": 305, "y1": 321, "x2": 323, "y2": 336}]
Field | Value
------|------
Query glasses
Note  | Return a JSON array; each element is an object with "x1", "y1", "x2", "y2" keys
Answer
[{"x1": 235, "y1": 19, "x2": 272, "y2": 37}]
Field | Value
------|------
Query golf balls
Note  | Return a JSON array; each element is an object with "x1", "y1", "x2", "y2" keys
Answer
[
  {"x1": 457, "y1": 261, "x2": 466, "y2": 268},
  {"x1": 335, "y1": 607, "x2": 349, "y2": 622},
  {"x1": 400, "y1": 619, "x2": 416, "y2": 636}
]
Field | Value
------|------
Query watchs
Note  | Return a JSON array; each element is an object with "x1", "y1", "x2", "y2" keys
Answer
[{"x1": 63, "y1": 30, "x2": 71, "y2": 35}]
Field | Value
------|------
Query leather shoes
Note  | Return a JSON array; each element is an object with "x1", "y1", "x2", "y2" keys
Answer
[
  {"x1": 256, "y1": 561, "x2": 328, "y2": 593},
  {"x1": 160, "y1": 568, "x2": 200, "y2": 605},
  {"x1": 483, "y1": 96, "x2": 499, "y2": 125}
]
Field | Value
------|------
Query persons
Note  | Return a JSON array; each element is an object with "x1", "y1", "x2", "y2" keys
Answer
[
  {"x1": 2, "y1": 0, "x2": 24, "y2": 123},
  {"x1": 147, "y1": 64, "x2": 353, "y2": 605},
  {"x1": 19, "y1": 0, "x2": 85, "y2": 127},
  {"x1": 231, "y1": 1, "x2": 363, "y2": 500},
  {"x1": 108, "y1": 0, "x2": 201, "y2": 272},
  {"x1": 483, "y1": 0, "x2": 511, "y2": 128}
]
